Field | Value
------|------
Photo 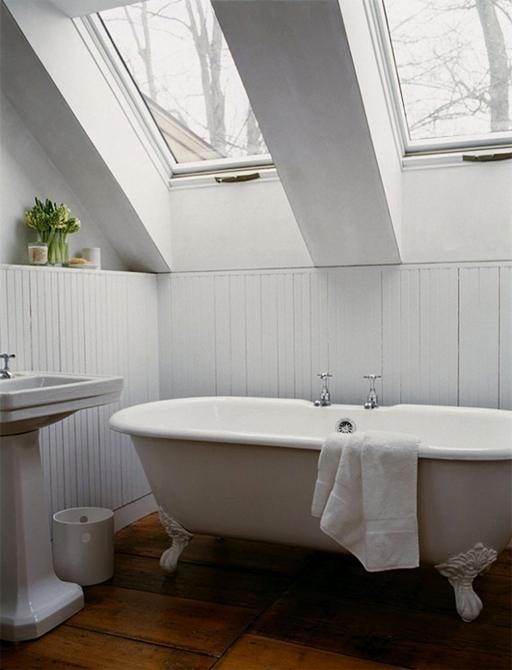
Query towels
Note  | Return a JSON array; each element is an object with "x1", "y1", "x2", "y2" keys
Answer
[{"x1": 311, "y1": 433, "x2": 422, "y2": 573}]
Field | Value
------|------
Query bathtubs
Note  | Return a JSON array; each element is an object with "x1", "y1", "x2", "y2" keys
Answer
[{"x1": 108, "y1": 396, "x2": 512, "y2": 565}]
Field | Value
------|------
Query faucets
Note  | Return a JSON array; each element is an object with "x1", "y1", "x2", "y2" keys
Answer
[
  {"x1": 0, "y1": 368, "x2": 13, "y2": 379},
  {"x1": 362, "y1": 374, "x2": 381, "y2": 409},
  {"x1": 313, "y1": 371, "x2": 334, "y2": 407}
]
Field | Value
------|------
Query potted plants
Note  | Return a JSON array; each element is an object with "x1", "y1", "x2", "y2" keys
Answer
[{"x1": 24, "y1": 197, "x2": 81, "y2": 267}]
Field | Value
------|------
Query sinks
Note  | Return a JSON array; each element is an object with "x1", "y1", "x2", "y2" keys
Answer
[{"x1": 0, "y1": 370, "x2": 125, "y2": 436}]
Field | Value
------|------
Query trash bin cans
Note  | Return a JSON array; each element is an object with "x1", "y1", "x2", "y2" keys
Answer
[{"x1": 53, "y1": 507, "x2": 115, "y2": 586}]
